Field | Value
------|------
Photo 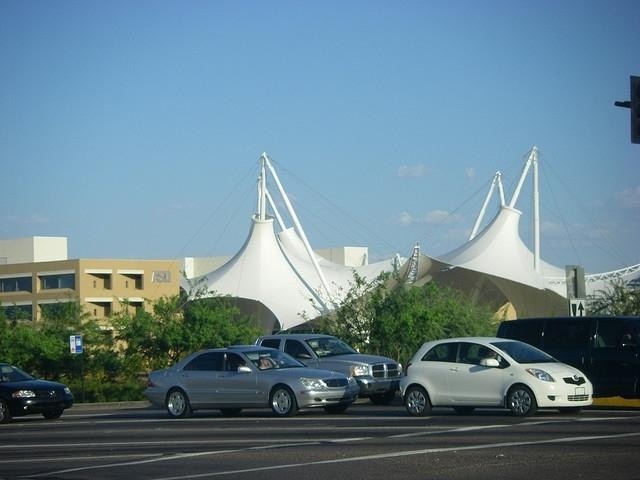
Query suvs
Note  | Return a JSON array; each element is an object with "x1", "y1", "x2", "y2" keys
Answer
[{"x1": 250, "y1": 333, "x2": 403, "y2": 405}]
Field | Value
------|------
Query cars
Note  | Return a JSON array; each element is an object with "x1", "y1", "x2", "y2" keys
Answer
[
  {"x1": 399, "y1": 334, "x2": 595, "y2": 416},
  {"x1": 1, "y1": 364, "x2": 74, "y2": 422},
  {"x1": 144, "y1": 345, "x2": 362, "y2": 418}
]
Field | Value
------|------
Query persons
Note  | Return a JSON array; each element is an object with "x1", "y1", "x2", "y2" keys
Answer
[{"x1": 258, "y1": 357, "x2": 273, "y2": 369}]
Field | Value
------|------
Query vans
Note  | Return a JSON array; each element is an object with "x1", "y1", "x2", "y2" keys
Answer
[{"x1": 496, "y1": 315, "x2": 639, "y2": 402}]
